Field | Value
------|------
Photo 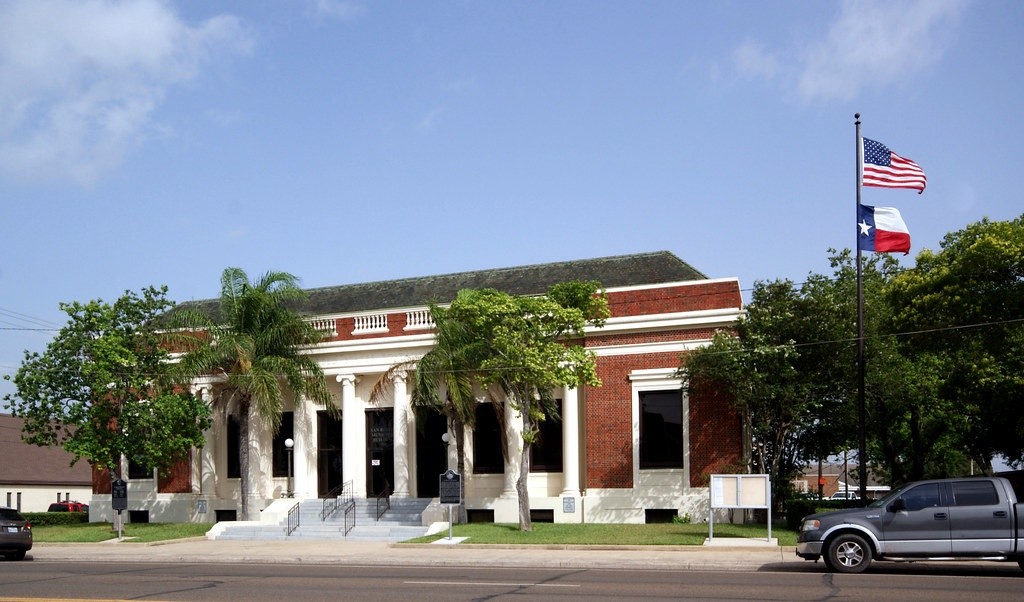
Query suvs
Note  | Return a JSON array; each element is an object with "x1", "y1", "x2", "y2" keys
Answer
[{"x1": 48, "y1": 501, "x2": 90, "y2": 512}]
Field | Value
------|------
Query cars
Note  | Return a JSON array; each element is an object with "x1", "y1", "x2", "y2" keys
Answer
[
  {"x1": 829, "y1": 491, "x2": 861, "y2": 501},
  {"x1": 0, "y1": 506, "x2": 33, "y2": 561}
]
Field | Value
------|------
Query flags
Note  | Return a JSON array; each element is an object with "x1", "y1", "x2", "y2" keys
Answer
[
  {"x1": 862, "y1": 138, "x2": 927, "y2": 194},
  {"x1": 857, "y1": 203, "x2": 911, "y2": 256}
]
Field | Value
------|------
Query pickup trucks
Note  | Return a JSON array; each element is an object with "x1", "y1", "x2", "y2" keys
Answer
[{"x1": 795, "y1": 475, "x2": 1024, "y2": 574}]
